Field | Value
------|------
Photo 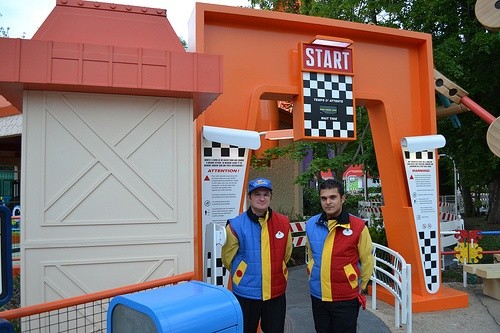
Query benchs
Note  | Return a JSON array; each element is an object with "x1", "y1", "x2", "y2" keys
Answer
[{"x1": 462, "y1": 263, "x2": 499, "y2": 300}]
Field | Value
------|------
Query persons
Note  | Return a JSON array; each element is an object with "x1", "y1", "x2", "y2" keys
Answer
[
  {"x1": 222, "y1": 179, "x2": 293, "y2": 333},
  {"x1": 304, "y1": 179, "x2": 374, "y2": 333}
]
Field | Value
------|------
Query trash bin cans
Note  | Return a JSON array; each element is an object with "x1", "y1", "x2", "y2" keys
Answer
[{"x1": 106, "y1": 278, "x2": 244, "y2": 333}]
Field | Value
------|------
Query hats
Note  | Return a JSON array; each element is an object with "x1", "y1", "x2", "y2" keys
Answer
[{"x1": 246, "y1": 179, "x2": 274, "y2": 195}]
